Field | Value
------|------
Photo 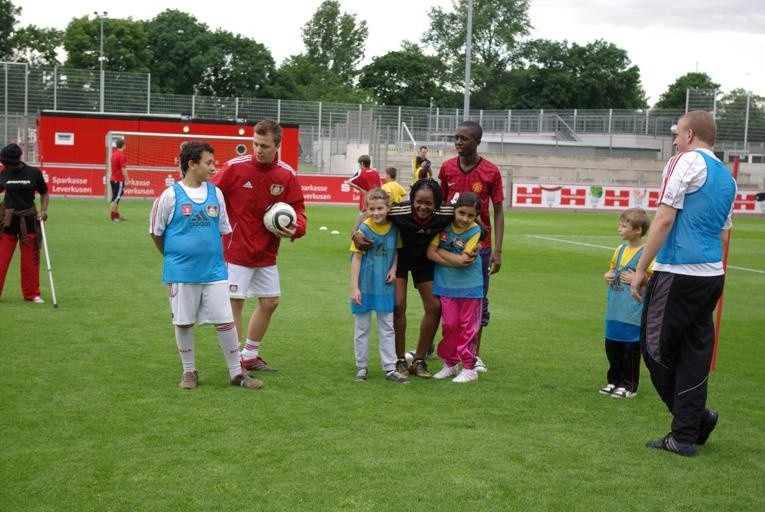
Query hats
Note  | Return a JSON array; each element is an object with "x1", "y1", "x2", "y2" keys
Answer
[{"x1": 0, "y1": 142, "x2": 23, "y2": 165}]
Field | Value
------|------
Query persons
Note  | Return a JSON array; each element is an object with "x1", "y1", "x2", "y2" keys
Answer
[
  {"x1": 0, "y1": 141, "x2": 51, "y2": 303},
  {"x1": 209, "y1": 118, "x2": 308, "y2": 373},
  {"x1": 346, "y1": 121, "x2": 504, "y2": 382},
  {"x1": 631, "y1": 109, "x2": 739, "y2": 457},
  {"x1": 598, "y1": 206, "x2": 654, "y2": 398},
  {"x1": 109, "y1": 139, "x2": 133, "y2": 223},
  {"x1": 148, "y1": 140, "x2": 266, "y2": 391}
]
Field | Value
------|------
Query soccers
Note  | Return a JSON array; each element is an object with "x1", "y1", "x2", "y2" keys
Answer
[{"x1": 264, "y1": 202, "x2": 297, "y2": 234}]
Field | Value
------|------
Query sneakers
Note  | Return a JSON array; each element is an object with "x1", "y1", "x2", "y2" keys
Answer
[
  {"x1": 111, "y1": 214, "x2": 126, "y2": 224},
  {"x1": 179, "y1": 367, "x2": 201, "y2": 390},
  {"x1": 240, "y1": 355, "x2": 279, "y2": 372},
  {"x1": 610, "y1": 386, "x2": 640, "y2": 399},
  {"x1": 646, "y1": 431, "x2": 699, "y2": 460},
  {"x1": 474, "y1": 356, "x2": 490, "y2": 372},
  {"x1": 229, "y1": 370, "x2": 263, "y2": 390},
  {"x1": 597, "y1": 381, "x2": 618, "y2": 395},
  {"x1": 32, "y1": 295, "x2": 45, "y2": 304},
  {"x1": 431, "y1": 361, "x2": 457, "y2": 379},
  {"x1": 698, "y1": 407, "x2": 719, "y2": 446},
  {"x1": 352, "y1": 357, "x2": 431, "y2": 384},
  {"x1": 449, "y1": 367, "x2": 480, "y2": 384}
]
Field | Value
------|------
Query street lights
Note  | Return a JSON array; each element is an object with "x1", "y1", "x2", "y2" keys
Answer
[{"x1": 94, "y1": 10, "x2": 108, "y2": 113}]
